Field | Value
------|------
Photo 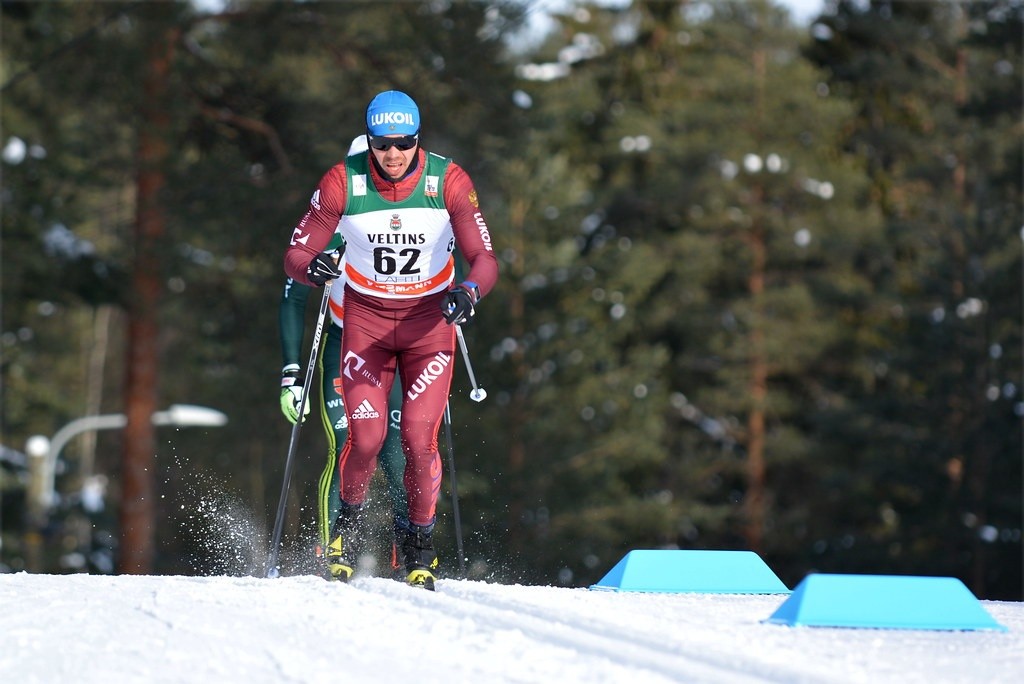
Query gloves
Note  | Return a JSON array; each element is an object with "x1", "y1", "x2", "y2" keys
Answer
[
  {"x1": 280, "y1": 372, "x2": 310, "y2": 427},
  {"x1": 439, "y1": 286, "x2": 477, "y2": 325},
  {"x1": 307, "y1": 253, "x2": 342, "y2": 286}
]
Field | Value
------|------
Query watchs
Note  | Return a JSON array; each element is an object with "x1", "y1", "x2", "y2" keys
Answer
[{"x1": 462, "y1": 280, "x2": 481, "y2": 304}]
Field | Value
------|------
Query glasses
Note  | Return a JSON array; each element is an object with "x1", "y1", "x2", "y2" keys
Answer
[{"x1": 368, "y1": 134, "x2": 420, "y2": 151}]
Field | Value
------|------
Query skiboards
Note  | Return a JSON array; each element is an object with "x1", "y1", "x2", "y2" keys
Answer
[{"x1": 338, "y1": 569, "x2": 436, "y2": 592}]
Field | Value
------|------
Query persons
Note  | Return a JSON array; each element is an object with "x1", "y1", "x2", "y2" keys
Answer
[{"x1": 280, "y1": 90, "x2": 498, "y2": 585}]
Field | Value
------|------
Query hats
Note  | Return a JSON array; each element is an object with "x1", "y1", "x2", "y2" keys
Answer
[{"x1": 367, "y1": 91, "x2": 419, "y2": 136}]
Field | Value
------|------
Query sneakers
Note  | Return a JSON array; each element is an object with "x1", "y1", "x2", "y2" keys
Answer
[
  {"x1": 401, "y1": 533, "x2": 439, "y2": 587},
  {"x1": 325, "y1": 519, "x2": 367, "y2": 582}
]
